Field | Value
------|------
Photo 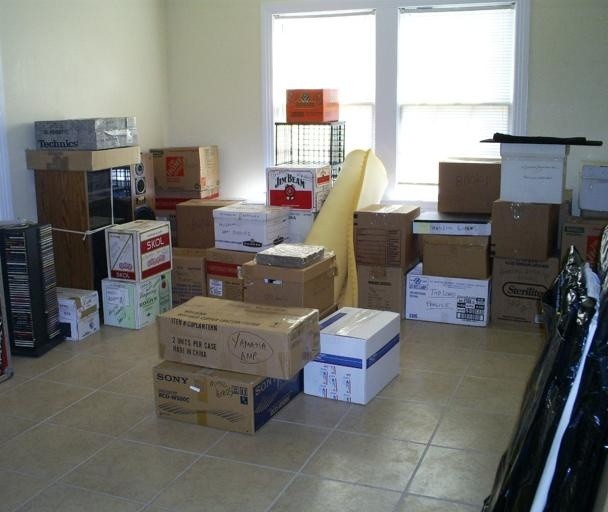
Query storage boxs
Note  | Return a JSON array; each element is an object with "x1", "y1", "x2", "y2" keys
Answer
[
  {"x1": 575, "y1": 160, "x2": 608, "y2": 213},
  {"x1": 353, "y1": 205, "x2": 420, "y2": 266},
  {"x1": 355, "y1": 261, "x2": 412, "y2": 314},
  {"x1": 175, "y1": 197, "x2": 245, "y2": 249},
  {"x1": 437, "y1": 157, "x2": 500, "y2": 215},
  {"x1": 206, "y1": 248, "x2": 257, "y2": 303},
  {"x1": 56, "y1": 286, "x2": 102, "y2": 343},
  {"x1": 151, "y1": 361, "x2": 302, "y2": 432},
  {"x1": 256, "y1": 244, "x2": 325, "y2": 269},
  {"x1": 488, "y1": 258, "x2": 558, "y2": 330},
  {"x1": 412, "y1": 211, "x2": 493, "y2": 238},
  {"x1": 106, "y1": 218, "x2": 173, "y2": 282},
  {"x1": 302, "y1": 307, "x2": 401, "y2": 407},
  {"x1": 285, "y1": 90, "x2": 341, "y2": 125},
  {"x1": 150, "y1": 143, "x2": 220, "y2": 209},
  {"x1": 489, "y1": 198, "x2": 557, "y2": 262},
  {"x1": 265, "y1": 162, "x2": 332, "y2": 212},
  {"x1": 243, "y1": 253, "x2": 339, "y2": 310},
  {"x1": 212, "y1": 201, "x2": 289, "y2": 254},
  {"x1": 34, "y1": 116, "x2": 136, "y2": 149},
  {"x1": 156, "y1": 295, "x2": 323, "y2": 381},
  {"x1": 404, "y1": 263, "x2": 492, "y2": 329},
  {"x1": 286, "y1": 209, "x2": 319, "y2": 243},
  {"x1": 154, "y1": 207, "x2": 178, "y2": 247},
  {"x1": 102, "y1": 269, "x2": 173, "y2": 331},
  {"x1": 558, "y1": 216, "x2": 606, "y2": 271},
  {"x1": 420, "y1": 232, "x2": 490, "y2": 279},
  {"x1": 171, "y1": 246, "x2": 207, "y2": 306},
  {"x1": 498, "y1": 142, "x2": 570, "y2": 205}
]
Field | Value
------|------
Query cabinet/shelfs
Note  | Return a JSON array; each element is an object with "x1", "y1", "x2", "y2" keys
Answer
[
  {"x1": 274, "y1": 120, "x2": 346, "y2": 182},
  {"x1": 23, "y1": 146, "x2": 141, "y2": 327},
  {"x1": 0, "y1": 222, "x2": 64, "y2": 359}
]
